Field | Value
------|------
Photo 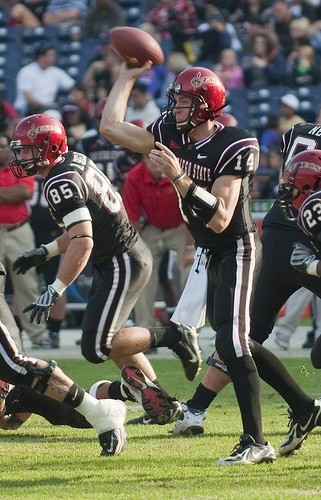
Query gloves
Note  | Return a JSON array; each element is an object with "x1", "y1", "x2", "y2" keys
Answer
[
  {"x1": 22, "y1": 285, "x2": 58, "y2": 324},
  {"x1": 11, "y1": 244, "x2": 49, "y2": 275}
]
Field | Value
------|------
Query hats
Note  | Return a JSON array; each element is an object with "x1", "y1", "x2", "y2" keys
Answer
[{"x1": 280, "y1": 93, "x2": 300, "y2": 113}]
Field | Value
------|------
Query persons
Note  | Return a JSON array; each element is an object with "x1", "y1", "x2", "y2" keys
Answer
[
  {"x1": 0, "y1": 99, "x2": 321, "y2": 456},
  {"x1": 0, "y1": 0, "x2": 321, "y2": 199},
  {"x1": 99, "y1": 60, "x2": 321, "y2": 465}
]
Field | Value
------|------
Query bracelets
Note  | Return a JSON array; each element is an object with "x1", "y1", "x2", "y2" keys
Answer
[{"x1": 173, "y1": 171, "x2": 187, "y2": 185}]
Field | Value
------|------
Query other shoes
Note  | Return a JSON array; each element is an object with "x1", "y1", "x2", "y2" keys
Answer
[{"x1": 32, "y1": 335, "x2": 60, "y2": 349}]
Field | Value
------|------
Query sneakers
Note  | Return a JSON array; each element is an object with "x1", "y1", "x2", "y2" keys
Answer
[
  {"x1": 278, "y1": 399, "x2": 321, "y2": 456},
  {"x1": 96, "y1": 397, "x2": 124, "y2": 456},
  {"x1": 125, "y1": 412, "x2": 164, "y2": 425},
  {"x1": 120, "y1": 364, "x2": 172, "y2": 425},
  {"x1": 169, "y1": 321, "x2": 202, "y2": 382},
  {"x1": 170, "y1": 401, "x2": 206, "y2": 436},
  {"x1": 216, "y1": 434, "x2": 276, "y2": 467}
]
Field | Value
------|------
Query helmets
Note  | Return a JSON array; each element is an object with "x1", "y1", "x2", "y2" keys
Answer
[
  {"x1": 166, "y1": 66, "x2": 226, "y2": 119},
  {"x1": 9, "y1": 114, "x2": 68, "y2": 170},
  {"x1": 94, "y1": 97, "x2": 106, "y2": 122},
  {"x1": 284, "y1": 149, "x2": 321, "y2": 210}
]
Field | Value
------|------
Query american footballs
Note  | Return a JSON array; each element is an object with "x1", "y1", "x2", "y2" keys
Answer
[{"x1": 110, "y1": 26, "x2": 164, "y2": 69}]
